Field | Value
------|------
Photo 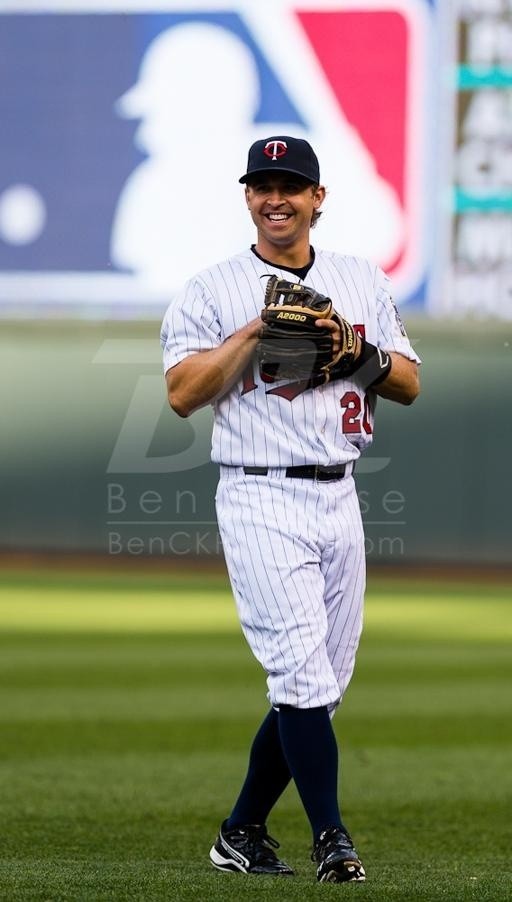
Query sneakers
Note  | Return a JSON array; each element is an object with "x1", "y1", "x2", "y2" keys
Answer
[
  {"x1": 310, "y1": 824, "x2": 367, "y2": 884},
  {"x1": 208, "y1": 819, "x2": 294, "y2": 877}
]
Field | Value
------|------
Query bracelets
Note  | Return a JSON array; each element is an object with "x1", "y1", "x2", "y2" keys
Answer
[{"x1": 346, "y1": 338, "x2": 393, "y2": 388}]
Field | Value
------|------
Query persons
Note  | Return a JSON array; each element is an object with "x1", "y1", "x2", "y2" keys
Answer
[{"x1": 156, "y1": 134, "x2": 423, "y2": 885}]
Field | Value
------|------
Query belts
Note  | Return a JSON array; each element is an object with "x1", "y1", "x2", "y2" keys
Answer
[{"x1": 243, "y1": 459, "x2": 356, "y2": 482}]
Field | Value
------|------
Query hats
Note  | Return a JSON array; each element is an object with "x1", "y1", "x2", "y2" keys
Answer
[{"x1": 238, "y1": 136, "x2": 321, "y2": 184}]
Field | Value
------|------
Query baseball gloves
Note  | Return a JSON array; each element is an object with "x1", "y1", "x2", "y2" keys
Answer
[{"x1": 258, "y1": 274, "x2": 361, "y2": 386}]
